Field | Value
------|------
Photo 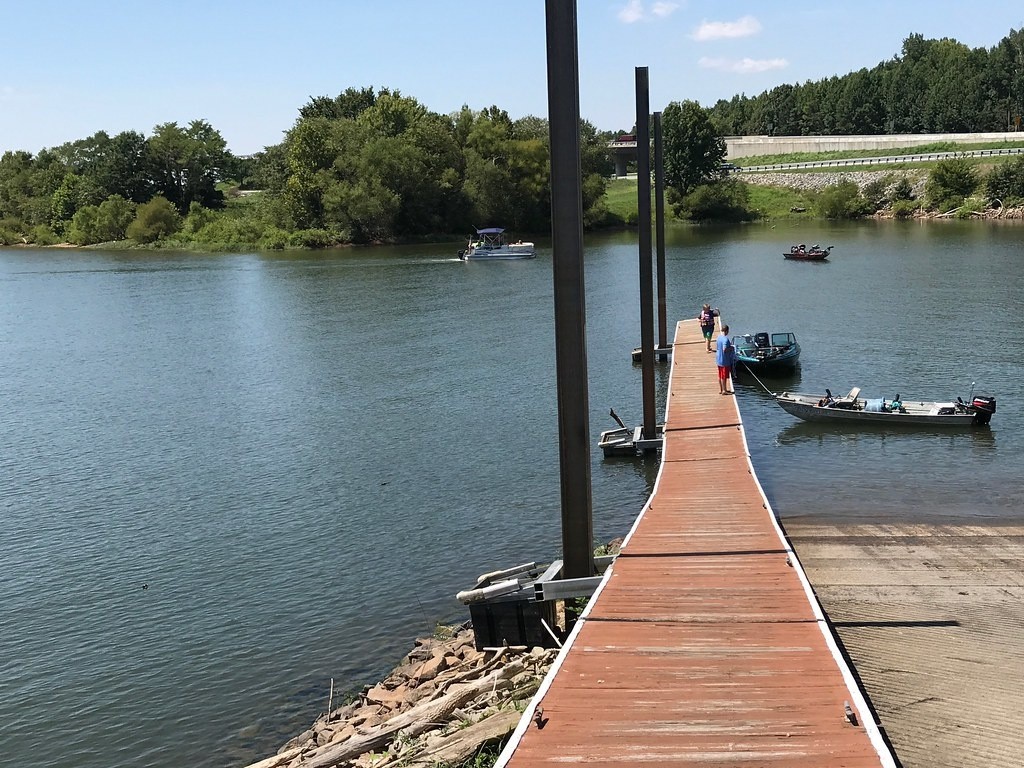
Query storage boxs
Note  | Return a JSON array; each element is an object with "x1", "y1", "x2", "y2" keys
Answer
[{"x1": 469, "y1": 600, "x2": 556, "y2": 652}]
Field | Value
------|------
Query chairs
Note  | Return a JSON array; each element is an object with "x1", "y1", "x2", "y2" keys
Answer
[
  {"x1": 833, "y1": 387, "x2": 861, "y2": 409},
  {"x1": 754, "y1": 332, "x2": 770, "y2": 353}
]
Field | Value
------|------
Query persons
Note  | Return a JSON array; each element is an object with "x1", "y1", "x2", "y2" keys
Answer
[
  {"x1": 476, "y1": 240, "x2": 482, "y2": 247},
  {"x1": 715, "y1": 324, "x2": 734, "y2": 395},
  {"x1": 698, "y1": 304, "x2": 720, "y2": 352},
  {"x1": 519, "y1": 240, "x2": 523, "y2": 245}
]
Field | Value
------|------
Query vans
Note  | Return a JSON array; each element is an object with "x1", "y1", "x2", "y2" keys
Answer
[{"x1": 720, "y1": 164, "x2": 743, "y2": 172}]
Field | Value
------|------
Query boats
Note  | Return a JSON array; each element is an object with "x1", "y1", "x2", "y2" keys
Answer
[
  {"x1": 729, "y1": 332, "x2": 802, "y2": 373},
  {"x1": 782, "y1": 244, "x2": 830, "y2": 259},
  {"x1": 775, "y1": 383, "x2": 997, "y2": 427}
]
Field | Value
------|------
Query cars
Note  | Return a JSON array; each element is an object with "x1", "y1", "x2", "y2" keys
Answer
[{"x1": 456, "y1": 229, "x2": 537, "y2": 261}]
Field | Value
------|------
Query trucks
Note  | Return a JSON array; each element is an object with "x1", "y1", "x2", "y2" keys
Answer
[{"x1": 617, "y1": 135, "x2": 636, "y2": 142}]
